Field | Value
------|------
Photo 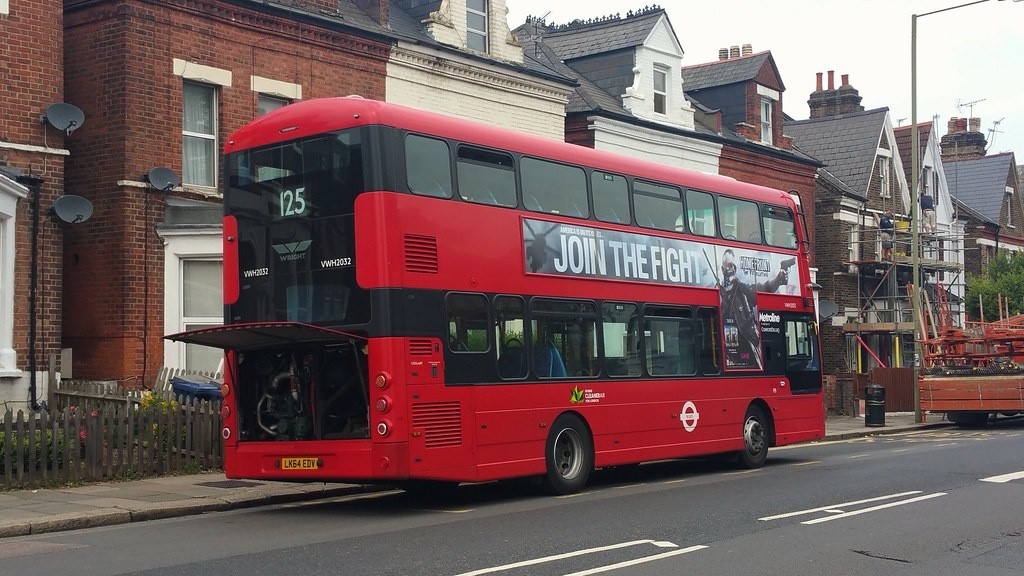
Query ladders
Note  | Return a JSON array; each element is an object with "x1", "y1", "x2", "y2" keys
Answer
[{"x1": 936, "y1": 283, "x2": 953, "y2": 330}]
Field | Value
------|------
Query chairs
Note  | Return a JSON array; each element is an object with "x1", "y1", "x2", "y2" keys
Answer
[
  {"x1": 497, "y1": 338, "x2": 526, "y2": 377},
  {"x1": 449, "y1": 335, "x2": 468, "y2": 351},
  {"x1": 572, "y1": 369, "x2": 590, "y2": 376},
  {"x1": 483, "y1": 186, "x2": 497, "y2": 203},
  {"x1": 533, "y1": 335, "x2": 567, "y2": 377},
  {"x1": 568, "y1": 201, "x2": 583, "y2": 218},
  {"x1": 528, "y1": 194, "x2": 542, "y2": 210},
  {"x1": 725, "y1": 234, "x2": 737, "y2": 239},
  {"x1": 748, "y1": 231, "x2": 760, "y2": 243},
  {"x1": 430, "y1": 178, "x2": 448, "y2": 198},
  {"x1": 644, "y1": 213, "x2": 655, "y2": 227},
  {"x1": 609, "y1": 206, "x2": 620, "y2": 223}
]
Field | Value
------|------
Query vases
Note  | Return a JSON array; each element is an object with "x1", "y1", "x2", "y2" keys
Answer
[
  {"x1": 76, "y1": 445, "x2": 85, "y2": 458},
  {"x1": 149, "y1": 450, "x2": 179, "y2": 472}
]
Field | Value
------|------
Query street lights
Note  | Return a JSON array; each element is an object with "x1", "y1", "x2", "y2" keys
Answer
[{"x1": 911, "y1": 0, "x2": 1024, "y2": 422}]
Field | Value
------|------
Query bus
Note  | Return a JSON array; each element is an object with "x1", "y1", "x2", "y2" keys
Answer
[{"x1": 159, "y1": 95, "x2": 825, "y2": 495}]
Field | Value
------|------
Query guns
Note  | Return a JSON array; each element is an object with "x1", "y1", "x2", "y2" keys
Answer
[{"x1": 779, "y1": 257, "x2": 795, "y2": 286}]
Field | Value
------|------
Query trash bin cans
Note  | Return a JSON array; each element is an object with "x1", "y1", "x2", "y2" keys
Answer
[
  {"x1": 864, "y1": 385, "x2": 887, "y2": 428},
  {"x1": 170, "y1": 373, "x2": 224, "y2": 456}
]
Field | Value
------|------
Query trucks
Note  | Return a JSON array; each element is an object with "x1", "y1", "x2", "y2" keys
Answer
[{"x1": 916, "y1": 282, "x2": 1024, "y2": 431}]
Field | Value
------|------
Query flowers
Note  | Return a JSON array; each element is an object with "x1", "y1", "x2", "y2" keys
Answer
[
  {"x1": 61, "y1": 406, "x2": 98, "y2": 445},
  {"x1": 141, "y1": 387, "x2": 189, "y2": 447}
]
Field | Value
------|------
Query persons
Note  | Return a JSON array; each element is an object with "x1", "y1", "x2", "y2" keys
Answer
[
  {"x1": 880, "y1": 210, "x2": 897, "y2": 262},
  {"x1": 917, "y1": 192, "x2": 936, "y2": 235},
  {"x1": 718, "y1": 249, "x2": 789, "y2": 369}
]
